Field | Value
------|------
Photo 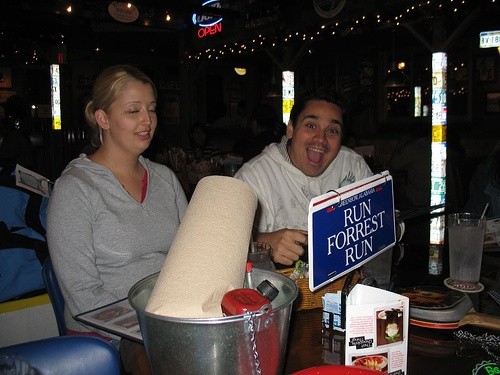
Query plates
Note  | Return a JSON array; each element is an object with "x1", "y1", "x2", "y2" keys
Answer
[
  {"x1": 444, "y1": 277, "x2": 484, "y2": 293},
  {"x1": 293, "y1": 365, "x2": 386, "y2": 375}
]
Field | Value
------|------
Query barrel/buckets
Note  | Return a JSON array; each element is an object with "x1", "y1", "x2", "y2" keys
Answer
[{"x1": 128, "y1": 267, "x2": 298, "y2": 375}]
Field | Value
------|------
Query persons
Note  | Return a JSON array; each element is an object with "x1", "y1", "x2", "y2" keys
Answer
[
  {"x1": 233, "y1": 91, "x2": 406, "y2": 270},
  {"x1": 45, "y1": 64, "x2": 190, "y2": 351},
  {"x1": 0, "y1": 91, "x2": 500, "y2": 220}
]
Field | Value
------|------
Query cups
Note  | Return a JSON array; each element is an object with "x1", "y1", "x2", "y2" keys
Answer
[{"x1": 448, "y1": 213, "x2": 486, "y2": 289}]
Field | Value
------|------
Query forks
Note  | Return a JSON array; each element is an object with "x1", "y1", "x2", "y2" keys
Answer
[{"x1": 487, "y1": 289, "x2": 500, "y2": 305}]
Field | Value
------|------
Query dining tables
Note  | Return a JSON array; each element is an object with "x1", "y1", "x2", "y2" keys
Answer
[{"x1": 118, "y1": 248, "x2": 500, "y2": 374}]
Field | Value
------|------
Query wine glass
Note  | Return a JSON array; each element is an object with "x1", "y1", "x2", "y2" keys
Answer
[{"x1": 171, "y1": 149, "x2": 181, "y2": 172}]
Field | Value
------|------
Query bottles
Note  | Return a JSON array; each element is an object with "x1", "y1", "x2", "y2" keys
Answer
[
  {"x1": 244, "y1": 263, "x2": 254, "y2": 289},
  {"x1": 243, "y1": 242, "x2": 275, "y2": 272},
  {"x1": 220, "y1": 280, "x2": 280, "y2": 317}
]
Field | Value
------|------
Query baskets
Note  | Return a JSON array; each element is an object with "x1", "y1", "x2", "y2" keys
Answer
[{"x1": 273, "y1": 260, "x2": 362, "y2": 309}]
